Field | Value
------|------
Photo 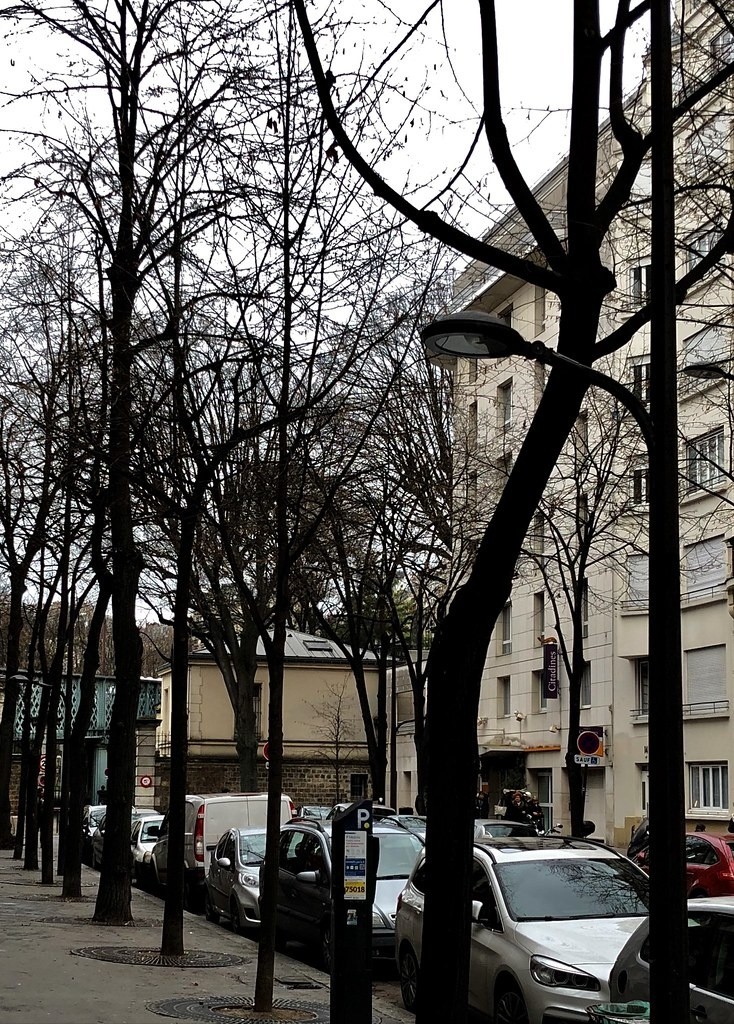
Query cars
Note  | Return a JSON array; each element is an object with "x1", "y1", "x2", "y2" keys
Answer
[
  {"x1": 291, "y1": 800, "x2": 397, "y2": 859},
  {"x1": 199, "y1": 827, "x2": 285, "y2": 932},
  {"x1": 469, "y1": 817, "x2": 540, "y2": 848},
  {"x1": 396, "y1": 835, "x2": 699, "y2": 1023},
  {"x1": 70, "y1": 803, "x2": 170, "y2": 886},
  {"x1": 379, "y1": 815, "x2": 444, "y2": 850},
  {"x1": 629, "y1": 827, "x2": 734, "y2": 900},
  {"x1": 612, "y1": 896, "x2": 734, "y2": 1024}
]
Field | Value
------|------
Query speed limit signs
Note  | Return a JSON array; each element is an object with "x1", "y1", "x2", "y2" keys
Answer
[{"x1": 40, "y1": 754, "x2": 52, "y2": 771}]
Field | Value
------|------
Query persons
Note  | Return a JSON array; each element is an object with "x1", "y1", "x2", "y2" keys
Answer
[
  {"x1": 476, "y1": 792, "x2": 490, "y2": 819},
  {"x1": 505, "y1": 792, "x2": 545, "y2": 827},
  {"x1": 96, "y1": 786, "x2": 107, "y2": 804}
]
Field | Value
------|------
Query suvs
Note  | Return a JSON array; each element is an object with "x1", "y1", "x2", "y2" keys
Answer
[{"x1": 258, "y1": 813, "x2": 434, "y2": 965}]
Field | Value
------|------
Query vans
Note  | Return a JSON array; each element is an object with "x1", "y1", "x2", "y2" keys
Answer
[{"x1": 147, "y1": 792, "x2": 296, "y2": 905}]
